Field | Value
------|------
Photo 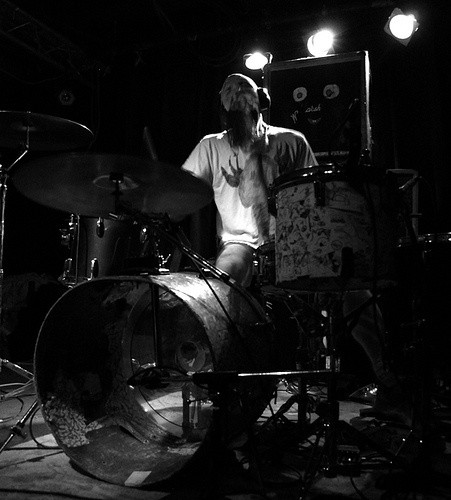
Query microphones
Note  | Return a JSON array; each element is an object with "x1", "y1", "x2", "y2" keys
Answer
[
  {"x1": 386, "y1": 176, "x2": 419, "y2": 208},
  {"x1": 130, "y1": 370, "x2": 169, "y2": 390}
]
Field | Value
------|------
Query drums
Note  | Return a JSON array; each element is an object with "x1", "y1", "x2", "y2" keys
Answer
[
  {"x1": 33, "y1": 270, "x2": 268, "y2": 488},
  {"x1": 61, "y1": 211, "x2": 184, "y2": 290},
  {"x1": 266, "y1": 162, "x2": 384, "y2": 294}
]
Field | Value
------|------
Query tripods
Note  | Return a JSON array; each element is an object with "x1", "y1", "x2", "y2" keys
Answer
[{"x1": 297, "y1": 199, "x2": 451, "y2": 500}]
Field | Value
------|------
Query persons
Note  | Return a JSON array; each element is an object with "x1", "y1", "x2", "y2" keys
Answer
[{"x1": 182, "y1": 73, "x2": 432, "y2": 434}]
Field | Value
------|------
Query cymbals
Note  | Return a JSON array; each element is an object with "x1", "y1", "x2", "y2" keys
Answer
[
  {"x1": 1, "y1": 108, "x2": 95, "y2": 154},
  {"x1": 11, "y1": 151, "x2": 215, "y2": 219}
]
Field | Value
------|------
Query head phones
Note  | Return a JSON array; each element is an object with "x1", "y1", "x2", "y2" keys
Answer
[{"x1": 220, "y1": 86, "x2": 271, "y2": 130}]
